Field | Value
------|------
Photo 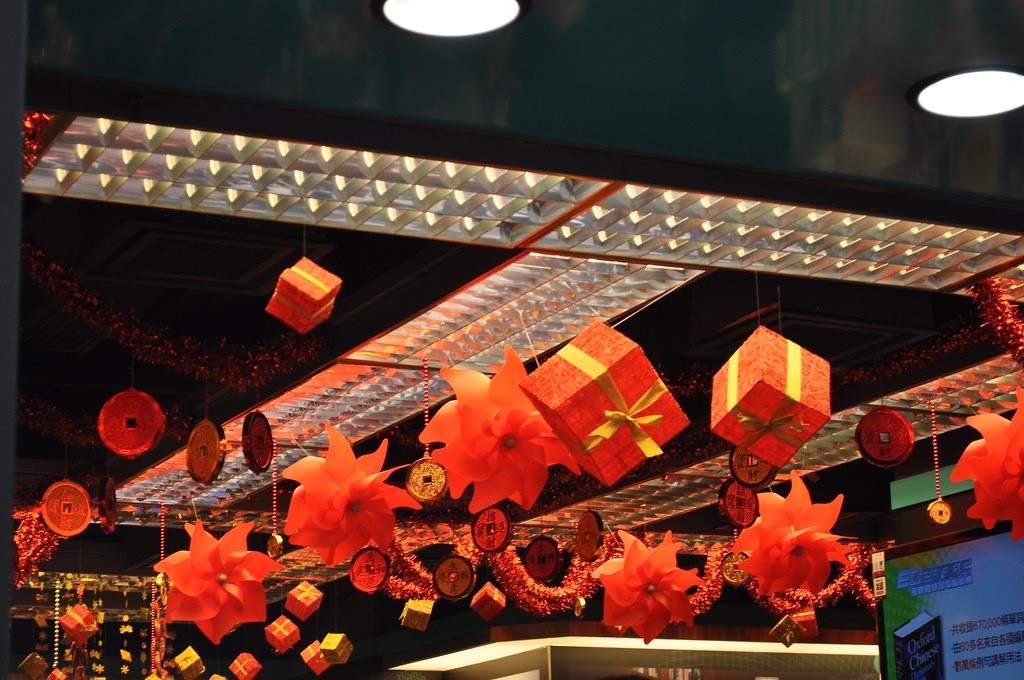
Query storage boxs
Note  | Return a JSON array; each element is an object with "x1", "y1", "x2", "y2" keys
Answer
[
  {"x1": 173, "y1": 646, "x2": 206, "y2": 680},
  {"x1": 470, "y1": 582, "x2": 507, "y2": 623},
  {"x1": 298, "y1": 640, "x2": 331, "y2": 676},
  {"x1": 319, "y1": 632, "x2": 354, "y2": 665},
  {"x1": 45, "y1": 668, "x2": 67, "y2": 680},
  {"x1": 793, "y1": 605, "x2": 820, "y2": 639},
  {"x1": 769, "y1": 615, "x2": 807, "y2": 649},
  {"x1": 264, "y1": 615, "x2": 301, "y2": 655},
  {"x1": 709, "y1": 326, "x2": 832, "y2": 471},
  {"x1": 59, "y1": 607, "x2": 99, "y2": 645},
  {"x1": 284, "y1": 580, "x2": 324, "y2": 622},
  {"x1": 228, "y1": 653, "x2": 263, "y2": 680},
  {"x1": 264, "y1": 257, "x2": 344, "y2": 338},
  {"x1": 398, "y1": 599, "x2": 436, "y2": 633},
  {"x1": 516, "y1": 321, "x2": 692, "y2": 491},
  {"x1": 18, "y1": 651, "x2": 48, "y2": 679}
]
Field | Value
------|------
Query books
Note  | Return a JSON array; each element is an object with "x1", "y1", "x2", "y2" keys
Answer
[{"x1": 892, "y1": 610, "x2": 944, "y2": 680}]
